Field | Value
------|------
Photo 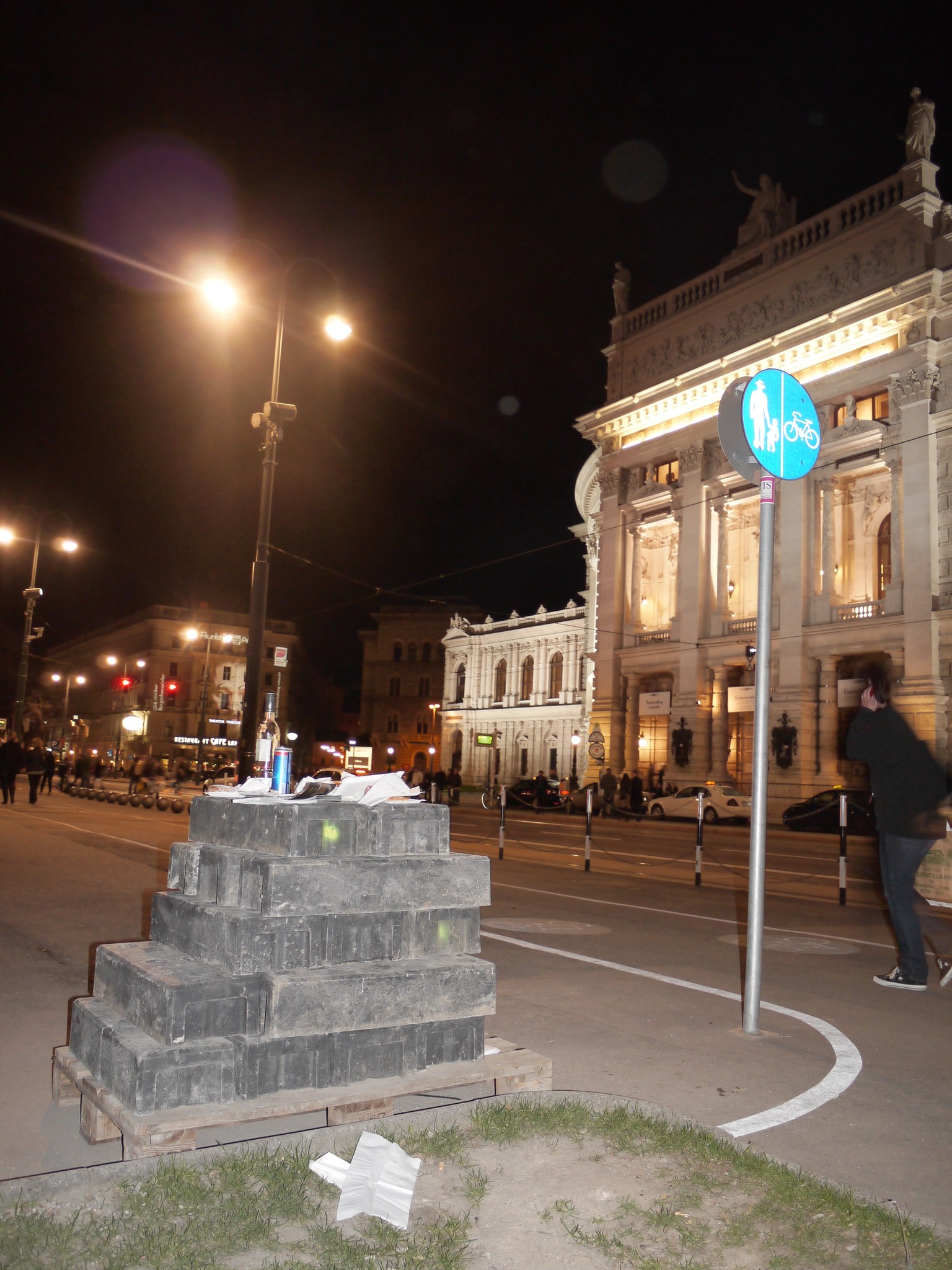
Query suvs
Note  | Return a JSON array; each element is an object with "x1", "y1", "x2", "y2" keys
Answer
[{"x1": 203, "y1": 766, "x2": 239, "y2": 795}]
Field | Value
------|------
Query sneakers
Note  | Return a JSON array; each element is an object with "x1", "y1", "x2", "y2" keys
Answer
[
  {"x1": 939, "y1": 961, "x2": 952, "y2": 987},
  {"x1": 872, "y1": 969, "x2": 926, "y2": 991}
]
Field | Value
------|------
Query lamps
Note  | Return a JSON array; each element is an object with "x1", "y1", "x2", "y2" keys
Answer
[
  {"x1": 640, "y1": 595, "x2": 648, "y2": 607},
  {"x1": 639, "y1": 731, "x2": 646, "y2": 749},
  {"x1": 570, "y1": 730, "x2": 580, "y2": 745},
  {"x1": 727, "y1": 579, "x2": 735, "y2": 593}
]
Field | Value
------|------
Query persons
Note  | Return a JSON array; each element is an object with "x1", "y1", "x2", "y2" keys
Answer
[
  {"x1": 627, "y1": 239, "x2": 894, "y2": 395},
  {"x1": 40, "y1": 750, "x2": 54, "y2": 795},
  {"x1": 611, "y1": 258, "x2": 632, "y2": 317},
  {"x1": 728, "y1": 170, "x2": 784, "y2": 236},
  {"x1": 850, "y1": 667, "x2": 951, "y2": 993},
  {"x1": 402, "y1": 759, "x2": 648, "y2": 825},
  {"x1": 0, "y1": 731, "x2": 20, "y2": 803},
  {"x1": 26, "y1": 738, "x2": 42, "y2": 804},
  {"x1": 905, "y1": 86, "x2": 936, "y2": 162},
  {"x1": 60, "y1": 744, "x2": 212, "y2": 797}
]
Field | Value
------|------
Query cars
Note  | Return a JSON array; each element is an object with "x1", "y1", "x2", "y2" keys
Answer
[
  {"x1": 499, "y1": 779, "x2": 561, "y2": 810},
  {"x1": 784, "y1": 787, "x2": 875, "y2": 834},
  {"x1": 648, "y1": 782, "x2": 754, "y2": 824},
  {"x1": 561, "y1": 782, "x2": 651, "y2": 816}
]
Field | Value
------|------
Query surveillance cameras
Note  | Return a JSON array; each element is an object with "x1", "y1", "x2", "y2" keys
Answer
[{"x1": 33, "y1": 627, "x2": 45, "y2": 632}]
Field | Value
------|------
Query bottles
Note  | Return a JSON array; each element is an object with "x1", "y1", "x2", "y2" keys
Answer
[{"x1": 254, "y1": 690, "x2": 281, "y2": 792}]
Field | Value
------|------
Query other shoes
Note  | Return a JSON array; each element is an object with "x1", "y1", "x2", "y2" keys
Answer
[
  {"x1": 47, "y1": 792, "x2": 50, "y2": 795},
  {"x1": 129, "y1": 791, "x2": 159, "y2": 799},
  {"x1": 71, "y1": 782, "x2": 104, "y2": 790},
  {"x1": 1, "y1": 800, "x2": 7, "y2": 803},
  {"x1": 40, "y1": 791, "x2": 42, "y2": 794},
  {"x1": 11, "y1": 800, "x2": 14, "y2": 803},
  {"x1": 536, "y1": 810, "x2": 545, "y2": 814},
  {"x1": 601, "y1": 813, "x2": 641, "y2": 822},
  {"x1": 448, "y1": 802, "x2": 460, "y2": 806}
]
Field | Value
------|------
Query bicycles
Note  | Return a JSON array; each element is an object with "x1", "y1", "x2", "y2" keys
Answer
[{"x1": 481, "y1": 784, "x2": 505, "y2": 808}]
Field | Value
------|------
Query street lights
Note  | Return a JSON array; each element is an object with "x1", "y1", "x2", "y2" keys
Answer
[
  {"x1": 107, "y1": 656, "x2": 147, "y2": 777},
  {"x1": 181, "y1": 627, "x2": 231, "y2": 782},
  {"x1": 201, "y1": 258, "x2": 352, "y2": 791},
  {"x1": 428, "y1": 703, "x2": 441, "y2": 782},
  {"x1": 51, "y1": 673, "x2": 87, "y2": 751}
]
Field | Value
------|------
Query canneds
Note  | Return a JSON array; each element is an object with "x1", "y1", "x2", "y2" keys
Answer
[{"x1": 272, "y1": 747, "x2": 293, "y2": 795}]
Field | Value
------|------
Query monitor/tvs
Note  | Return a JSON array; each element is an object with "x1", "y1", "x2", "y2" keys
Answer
[{"x1": 474, "y1": 733, "x2": 495, "y2": 747}]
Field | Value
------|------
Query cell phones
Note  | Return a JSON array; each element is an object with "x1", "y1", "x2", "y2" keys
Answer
[{"x1": 870, "y1": 688, "x2": 874, "y2": 697}]
